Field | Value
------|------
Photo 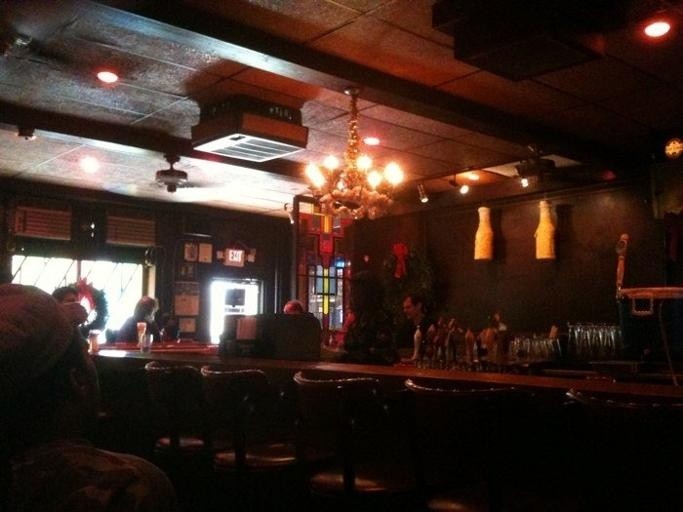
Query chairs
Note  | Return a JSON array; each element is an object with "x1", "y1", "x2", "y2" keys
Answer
[
  {"x1": 292, "y1": 371, "x2": 400, "y2": 510},
  {"x1": 145, "y1": 362, "x2": 200, "y2": 510},
  {"x1": 201, "y1": 365, "x2": 296, "y2": 508},
  {"x1": 564, "y1": 386, "x2": 681, "y2": 511},
  {"x1": 401, "y1": 377, "x2": 538, "y2": 511}
]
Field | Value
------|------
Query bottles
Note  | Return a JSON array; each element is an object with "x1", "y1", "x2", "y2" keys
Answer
[{"x1": 413, "y1": 316, "x2": 488, "y2": 367}]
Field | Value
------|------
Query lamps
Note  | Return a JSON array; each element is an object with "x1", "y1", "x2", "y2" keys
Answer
[
  {"x1": 415, "y1": 183, "x2": 428, "y2": 203},
  {"x1": 302, "y1": 87, "x2": 403, "y2": 221}
]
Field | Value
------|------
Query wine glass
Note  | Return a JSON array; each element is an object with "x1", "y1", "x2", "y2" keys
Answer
[{"x1": 566, "y1": 320, "x2": 624, "y2": 360}]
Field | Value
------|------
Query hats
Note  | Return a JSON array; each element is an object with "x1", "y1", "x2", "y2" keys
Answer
[{"x1": 0, "y1": 283, "x2": 87, "y2": 398}]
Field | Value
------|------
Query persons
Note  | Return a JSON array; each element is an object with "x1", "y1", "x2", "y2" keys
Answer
[
  {"x1": 282, "y1": 299, "x2": 305, "y2": 314},
  {"x1": 51, "y1": 284, "x2": 80, "y2": 307},
  {"x1": 339, "y1": 269, "x2": 401, "y2": 364},
  {"x1": 115, "y1": 295, "x2": 162, "y2": 344},
  {"x1": 397, "y1": 295, "x2": 443, "y2": 346},
  {"x1": 0, "y1": 282, "x2": 182, "y2": 512}
]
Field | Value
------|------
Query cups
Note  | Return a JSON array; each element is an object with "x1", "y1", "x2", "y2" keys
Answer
[
  {"x1": 141, "y1": 334, "x2": 153, "y2": 354},
  {"x1": 136, "y1": 322, "x2": 147, "y2": 347},
  {"x1": 88, "y1": 330, "x2": 100, "y2": 355},
  {"x1": 510, "y1": 336, "x2": 560, "y2": 361}
]
